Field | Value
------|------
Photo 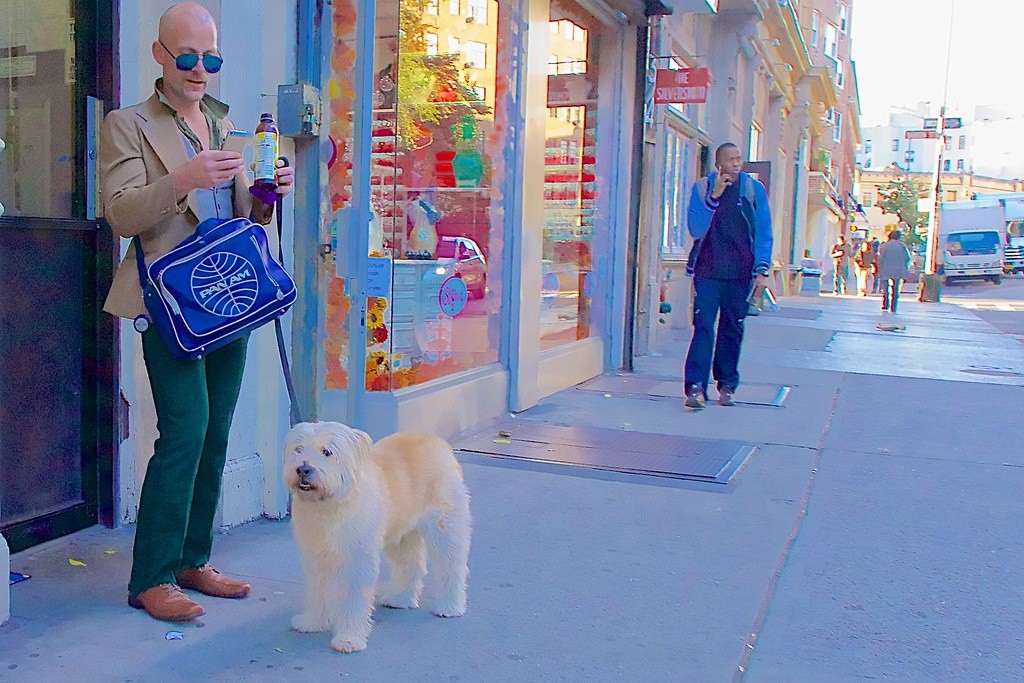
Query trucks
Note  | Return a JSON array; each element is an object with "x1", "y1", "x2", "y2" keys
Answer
[
  {"x1": 938, "y1": 199, "x2": 1005, "y2": 286},
  {"x1": 971, "y1": 192, "x2": 1024, "y2": 273}
]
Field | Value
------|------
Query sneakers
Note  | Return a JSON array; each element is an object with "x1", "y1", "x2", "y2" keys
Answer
[
  {"x1": 684, "y1": 381, "x2": 706, "y2": 408},
  {"x1": 719, "y1": 383, "x2": 735, "y2": 406}
]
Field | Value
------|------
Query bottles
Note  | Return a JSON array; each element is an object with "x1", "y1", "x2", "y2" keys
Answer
[{"x1": 253, "y1": 113, "x2": 279, "y2": 191}]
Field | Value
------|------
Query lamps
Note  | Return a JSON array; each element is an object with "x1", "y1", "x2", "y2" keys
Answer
[{"x1": 761, "y1": 38, "x2": 809, "y2": 107}]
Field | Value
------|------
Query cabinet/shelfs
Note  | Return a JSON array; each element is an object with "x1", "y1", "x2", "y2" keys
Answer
[{"x1": 382, "y1": 258, "x2": 456, "y2": 372}]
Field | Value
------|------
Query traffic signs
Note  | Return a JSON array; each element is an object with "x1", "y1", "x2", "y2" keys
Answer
[{"x1": 922, "y1": 117, "x2": 962, "y2": 129}]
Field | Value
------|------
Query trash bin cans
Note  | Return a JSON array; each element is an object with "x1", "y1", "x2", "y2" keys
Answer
[{"x1": 919, "y1": 274, "x2": 941, "y2": 303}]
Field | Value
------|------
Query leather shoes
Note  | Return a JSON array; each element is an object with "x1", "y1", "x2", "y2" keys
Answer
[
  {"x1": 128, "y1": 583, "x2": 206, "y2": 622},
  {"x1": 178, "y1": 562, "x2": 251, "y2": 599}
]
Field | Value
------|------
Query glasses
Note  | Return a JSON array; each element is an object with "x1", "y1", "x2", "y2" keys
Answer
[{"x1": 158, "y1": 39, "x2": 224, "y2": 74}]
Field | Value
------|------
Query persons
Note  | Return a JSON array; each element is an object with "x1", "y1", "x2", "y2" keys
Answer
[
  {"x1": 830, "y1": 236, "x2": 886, "y2": 296},
  {"x1": 99, "y1": 2, "x2": 294, "y2": 622},
  {"x1": 684, "y1": 143, "x2": 773, "y2": 409},
  {"x1": 877, "y1": 230, "x2": 910, "y2": 313}
]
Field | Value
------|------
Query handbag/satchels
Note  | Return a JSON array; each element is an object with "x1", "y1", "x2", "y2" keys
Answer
[{"x1": 143, "y1": 217, "x2": 298, "y2": 361}]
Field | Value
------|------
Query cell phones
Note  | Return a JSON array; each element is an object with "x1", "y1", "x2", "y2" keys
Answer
[{"x1": 221, "y1": 130, "x2": 248, "y2": 156}]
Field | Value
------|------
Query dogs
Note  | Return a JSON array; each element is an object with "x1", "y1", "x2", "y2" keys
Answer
[{"x1": 283, "y1": 420, "x2": 474, "y2": 653}]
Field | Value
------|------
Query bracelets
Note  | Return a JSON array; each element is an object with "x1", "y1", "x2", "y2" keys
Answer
[{"x1": 760, "y1": 270, "x2": 769, "y2": 277}]
